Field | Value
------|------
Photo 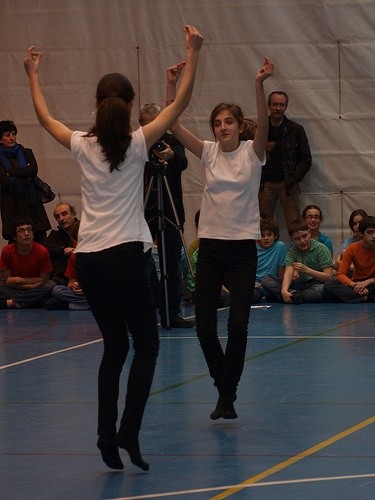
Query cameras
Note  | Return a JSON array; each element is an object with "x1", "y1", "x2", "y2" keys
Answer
[{"x1": 150, "y1": 139, "x2": 167, "y2": 164}]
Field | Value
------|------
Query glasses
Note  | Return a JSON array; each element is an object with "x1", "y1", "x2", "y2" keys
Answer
[
  {"x1": 14, "y1": 228, "x2": 33, "y2": 235},
  {"x1": 305, "y1": 214, "x2": 321, "y2": 220}
]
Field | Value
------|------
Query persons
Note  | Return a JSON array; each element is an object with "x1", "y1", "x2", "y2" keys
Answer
[
  {"x1": 22, "y1": 25, "x2": 204, "y2": 472},
  {"x1": 0, "y1": 216, "x2": 56, "y2": 309},
  {"x1": 259, "y1": 91, "x2": 312, "y2": 228},
  {"x1": 43, "y1": 203, "x2": 289, "y2": 310},
  {"x1": 166, "y1": 56, "x2": 275, "y2": 419},
  {"x1": 0, "y1": 120, "x2": 52, "y2": 243},
  {"x1": 137, "y1": 103, "x2": 197, "y2": 329},
  {"x1": 302, "y1": 205, "x2": 339, "y2": 277},
  {"x1": 325, "y1": 216, "x2": 375, "y2": 305},
  {"x1": 336, "y1": 209, "x2": 368, "y2": 279},
  {"x1": 261, "y1": 218, "x2": 334, "y2": 305},
  {"x1": 239, "y1": 119, "x2": 274, "y2": 156}
]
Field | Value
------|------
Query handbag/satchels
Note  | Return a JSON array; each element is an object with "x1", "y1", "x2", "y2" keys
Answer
[{"x1": 24, "y1": 148, "x2": 56, "y2": 204}]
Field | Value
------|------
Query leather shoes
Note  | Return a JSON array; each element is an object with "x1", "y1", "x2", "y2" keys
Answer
[{"x1": 161, "y1": 315, "x2": 195, "y2": 328}]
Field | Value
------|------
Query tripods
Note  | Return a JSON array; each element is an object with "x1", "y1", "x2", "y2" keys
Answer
[{"x1": 144, "y1": 165, "x2": 195, "y2": 330}]
